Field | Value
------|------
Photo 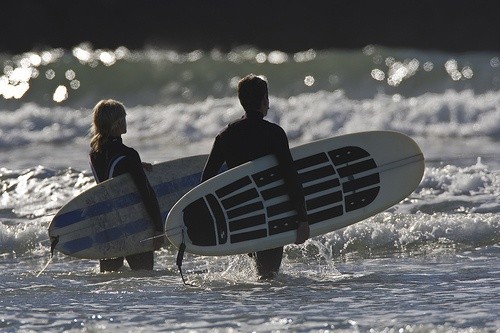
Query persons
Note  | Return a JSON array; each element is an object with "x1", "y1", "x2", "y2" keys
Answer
[
  {"x1": 88, "y1": 98, "x2": 166, "y2": 275},
  {"x1": 201, "y1": 73, "x2": 310, "y2": 282}
]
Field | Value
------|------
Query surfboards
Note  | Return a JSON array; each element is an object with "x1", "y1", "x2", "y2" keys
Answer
[
  {"x1": 165, "y1": 130, "x2": 425, "y2": 256},
  {"x1": 47, "y1": 153, "x2": 228, "y2": 260}
]
